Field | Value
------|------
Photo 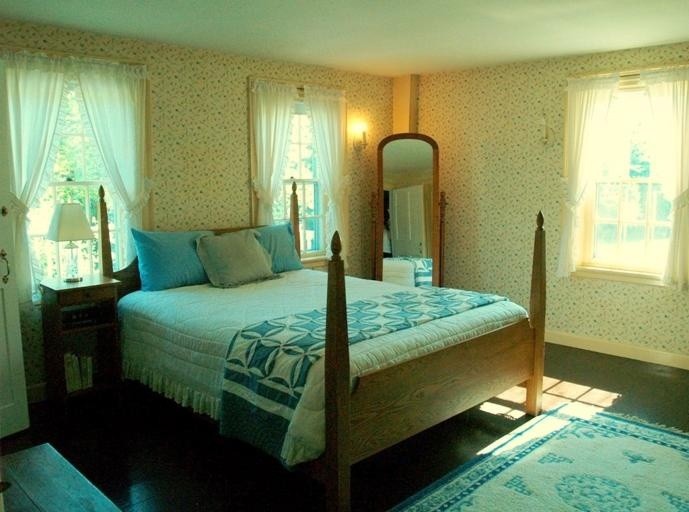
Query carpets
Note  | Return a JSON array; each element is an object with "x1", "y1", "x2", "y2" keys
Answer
[{"x1": 384, "y1": 397, "x2": 685, "y2": 510}]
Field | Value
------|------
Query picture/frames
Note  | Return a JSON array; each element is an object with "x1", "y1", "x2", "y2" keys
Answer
[{"x1": 374, "y1": 133, "x2": 442, "y2": 288}]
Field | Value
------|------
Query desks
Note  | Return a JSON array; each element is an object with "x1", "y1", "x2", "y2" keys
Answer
[{"x1": 2, "y1": 437, "x2": 121, "y2": 512}]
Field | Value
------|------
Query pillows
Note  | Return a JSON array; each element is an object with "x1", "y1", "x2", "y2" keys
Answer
[
  {"x1": 130, "y1": 226, "x2": 214, "y2": 293},
  {"x1": 250, "y1": 218, "x2": 304, "y2": 276},
  {"x1": 191, "y1": 226, "x2": 278, "y2": 287}
]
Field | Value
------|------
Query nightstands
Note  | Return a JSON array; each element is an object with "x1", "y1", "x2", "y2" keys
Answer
[{"x1": 38, "y1": 270, "x2": 120, "y2": 412}]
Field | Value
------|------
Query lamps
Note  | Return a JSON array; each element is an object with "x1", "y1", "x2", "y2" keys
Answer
[
  {"x1": 43, "y1": 200, "x2": 102, "y2": 283},
  {"x1": 350, "y1": 119, "x2": 371, "y2": 153}
]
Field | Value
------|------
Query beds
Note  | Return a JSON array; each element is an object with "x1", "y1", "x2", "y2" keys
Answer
[
  {"x1": 97, "y1": 174, "x2": 548, "y2": 512},
  {"x1": 383, "y1": 256, "x2": 433, "y2": 288}
]
